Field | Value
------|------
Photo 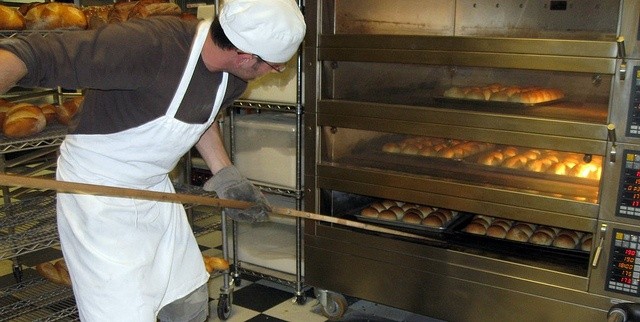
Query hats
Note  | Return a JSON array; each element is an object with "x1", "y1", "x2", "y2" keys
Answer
[{"x1": 218, "y1": 0, "x2": 307, "y2": 66}]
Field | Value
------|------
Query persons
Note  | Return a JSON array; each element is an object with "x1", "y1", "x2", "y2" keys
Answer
[{"x1": 0, "y1": 1, "x2": 307, "y2": 321}]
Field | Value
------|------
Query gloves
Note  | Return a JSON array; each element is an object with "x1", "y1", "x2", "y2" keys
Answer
[{"x1": 203, "y1": 164, "x2": 273, "y2": 224}]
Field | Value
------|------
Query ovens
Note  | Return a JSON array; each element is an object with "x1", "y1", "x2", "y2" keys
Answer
[{"x1": 303, "y1": 0, "x2": 640, "y2": 322}]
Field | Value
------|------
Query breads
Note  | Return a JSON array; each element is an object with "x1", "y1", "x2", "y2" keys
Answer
[
  {"x1": 210, "y1": 253, "x2": 230, "y2": 269},
  {"x1": 51, "y1": 258, "x2": 65, "y2": 272},
  {"x1": 128, "y1": 3, "x2": 182, "y2": 21},
  {"x1": 1, "y1": 4, "x2": 22, "y2": 30},
  {"x1": 3, "y1": 102, "x2": 47, "y2": 138},
  {"x1": 465, "y1": 215, "x2": 593, "y2": 253},
  {"x1": 80, "y1": 4, "x2": 105, "y2": 23},
  {"x1": 0, "y1": 99, "x2": 16, "y2": 127},
  {"x1": 17, "y1": 3, "x2": 45, "y2": 15},
  {"x1": 54, "y1": 265, "x2": 71, "y2": 290},
  {"x1": 39, "y1": 102, "x2": 57, "y2": 122},
  {"x1": 444, "y1": 81, "x2": 565, "y2": 105},
  {"x1": 360, "y1": 199, "x2": 458, "y2": 230},
  {"x1": 200, "y1": 253, "x2": 215, "y2": 277},
  {"x1": 107, "y1": 3, "x2": 137, "y2": 24},
  {"x1": 38, "y1": 262, "x2": 57, "y2": 280},
  {"x1": 56, "y1": 96, "x2": 87, "y2": 124},
  {"x1": 89, "y1": 8, "x2": 115, "y2": 29},
  {"x1": 24, "y1": 2, "x2": 88, "y2": 33},
  {"x1": 381, "y1": 135, "x2": 603, "y2": 184}
]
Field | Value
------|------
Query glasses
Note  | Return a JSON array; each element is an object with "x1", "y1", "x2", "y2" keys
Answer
[{"x1": 252, "y1": 54, "x2": 291, "y2": 73}]
